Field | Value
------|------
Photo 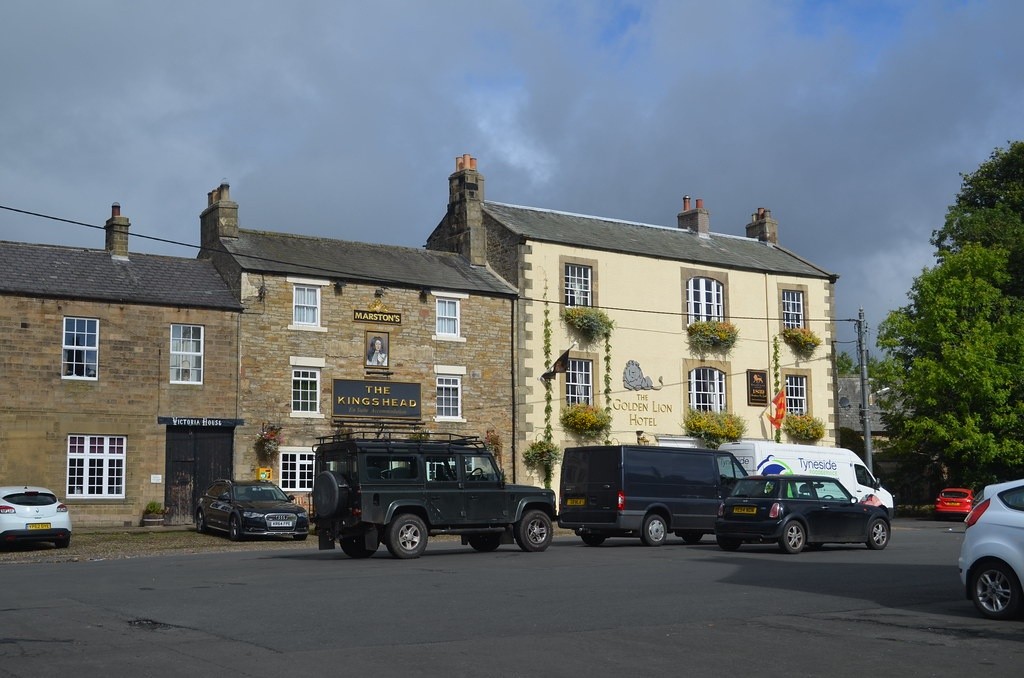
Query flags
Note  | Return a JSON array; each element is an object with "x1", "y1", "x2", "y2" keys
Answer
[
  {"x1": 542, "y1": 349, "x2": 570, "y2": 380},
  {"x1": 764, "y1": 388, "x2": 786, "y2": 431}
]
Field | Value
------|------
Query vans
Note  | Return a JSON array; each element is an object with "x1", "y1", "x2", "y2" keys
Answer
[
  {"x1": 558, "y1": 444, "x2": 749, "y2": 547},
  {"x1": 716, "y1": 441, "x2": 894, "y2": 522}
]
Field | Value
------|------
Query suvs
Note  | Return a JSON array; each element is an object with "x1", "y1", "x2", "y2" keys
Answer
[{"x1": 311, "y1": 431, "x2": 559, "y2": 558}]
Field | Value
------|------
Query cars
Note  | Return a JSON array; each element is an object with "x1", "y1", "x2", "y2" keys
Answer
[
  {"x1": 935, "y1": 488, "x2": 975, "y2": 520},
  {"x1": 0, "y1": 485, "x2": 71, "y2": 548},
  {"x1": 195, "y1": 478, "x2": 310, "y2": 540},
  {"x1": 957, "y1": 478, "x2": 1024, "y2": 620},
  {"x1": 715, "y1": 473, "x2": 891, "y2": 554}
]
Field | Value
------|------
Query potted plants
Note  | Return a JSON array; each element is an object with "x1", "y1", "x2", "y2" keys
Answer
[{"x1": 141, "y1": 500, "x2": 165, "y2": 526}]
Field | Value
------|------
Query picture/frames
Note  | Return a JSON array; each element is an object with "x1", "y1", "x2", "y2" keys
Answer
[{"x1": 364, "y1": 329, "x2": 391, "y2": 369}]
